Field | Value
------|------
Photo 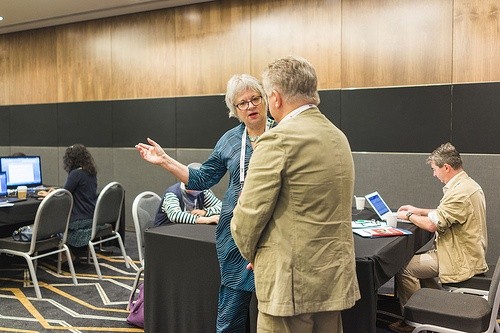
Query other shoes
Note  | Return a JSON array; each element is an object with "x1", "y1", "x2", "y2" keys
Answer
[
  {"x1": 73, "y1": 258, "x2": 81, "y2": 272},
  {"x1": 55, "y1": 261, "x2": 70, "y2": 272},
  {"x1": 388, "y1": 321, "x2": 422, "y2": 333}
]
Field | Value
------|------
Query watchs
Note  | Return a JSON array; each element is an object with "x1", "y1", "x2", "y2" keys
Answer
[{"x1": 406, "y1": 212, "x2": 413, "y2": 220}]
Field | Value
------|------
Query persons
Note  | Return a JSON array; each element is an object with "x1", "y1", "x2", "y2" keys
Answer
[
  {"x1": 154, "y1": 162, "x2": 222, "y2": 227},
  {"x1": 38, "y1": 144, "x2": 99, "y2": 264},
  {"x1": 135, "y1": 73, "x2": 279, "y2": 333},
  {"x1": 389, "y1": 143, "x2": 489, "y2": 333},
  {"x1": 230, "y1": 56, "x2": 361, "y2": 333}
]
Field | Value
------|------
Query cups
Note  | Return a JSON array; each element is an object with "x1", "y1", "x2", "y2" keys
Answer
[
  {"x1": 355, "y1": 196, "x2": 365, "y2": 210},
  {"x1": 386, "y1": 212, "x2": 397, "y2": 228},
  {"x1": 17, "y1": 186, "x2": 27, "y2": 200}
]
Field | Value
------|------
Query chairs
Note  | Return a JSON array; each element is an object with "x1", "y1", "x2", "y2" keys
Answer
[
  {"x1": 0, "y1": 188, "x2": 78, "y2": 299},
  {"x1": 443, "y1": 264, "x2": 496, "y2": 295},
  {"x1": 404, "y1": 255, "x2": 500, "y2": 333},
  {"x1": 126, "y1": 190, "x2": 162, "y2": 311},
  {"x1": 56, "y1": 181, "x2": 131, "y2": 279}
]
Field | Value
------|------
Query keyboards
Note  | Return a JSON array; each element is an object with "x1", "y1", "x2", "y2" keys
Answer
[{"x1": 30, "y1": 194, "x2": 44, "y2": 198}]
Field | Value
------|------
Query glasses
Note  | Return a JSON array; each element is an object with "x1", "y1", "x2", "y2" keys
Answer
[{"x1": 234, "y1": 96, "x2": 262, "y2": 111}]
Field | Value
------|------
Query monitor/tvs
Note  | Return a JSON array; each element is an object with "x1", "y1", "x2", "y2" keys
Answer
[{"x1": 0, "y1": 155, "x2": 42, "y2": 195}]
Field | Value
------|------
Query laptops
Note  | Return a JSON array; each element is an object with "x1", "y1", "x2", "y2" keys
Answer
[{"x1": 364, "y1": 191, "x2": 412, "y2": 223}]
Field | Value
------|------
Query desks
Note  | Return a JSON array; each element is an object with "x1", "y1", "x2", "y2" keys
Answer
[
  {"x1": 0, "y1": 196, "x2": 42, "y2": 228},
  {"x1": 143, "y1": 207, "x2": 435, "y2": 333}
]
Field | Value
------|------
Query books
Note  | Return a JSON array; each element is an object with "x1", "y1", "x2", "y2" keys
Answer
[{"x1": 352, "y1": 219, "x2": 413, "y2": 238}]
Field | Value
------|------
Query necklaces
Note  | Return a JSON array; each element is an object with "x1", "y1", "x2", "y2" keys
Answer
[{"x1": 249, "y1": 137, "x2": 258, "y2": 142}]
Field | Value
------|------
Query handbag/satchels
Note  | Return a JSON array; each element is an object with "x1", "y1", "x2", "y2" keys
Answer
[
  {"x1": 126, "y1": 267, "x2": 144, "y2": 329},
  {"x1": 12, "y1": 225, "x2": 56, "y2": 241}
]
